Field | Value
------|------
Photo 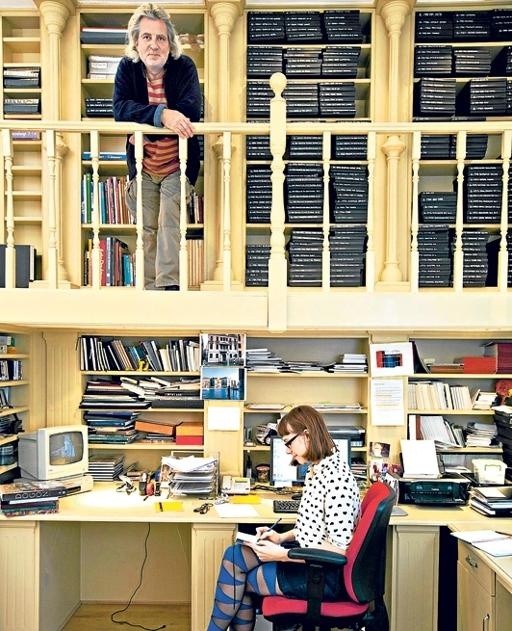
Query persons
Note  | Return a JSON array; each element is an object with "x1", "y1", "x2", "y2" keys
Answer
[
  {"x1": 206, "y1": 404, "x2": 361, "y2": 631},
  {"x1": 111, "y1": 3, "x2": 203, "y2": 289}
]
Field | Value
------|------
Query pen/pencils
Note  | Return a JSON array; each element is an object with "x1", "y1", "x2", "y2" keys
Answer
[
  {"x1": 257, "y1": 518, "x2": 282, "y2": 542},
  {"x1": 494, "y1": 531, "x2": 512, "y2": 537},
  {"x1": 158, "y1": 502, "x2": 163, "y2": 511},
  {"x1": 143, "y1": 496, "x2": 148, "y2": 501}
]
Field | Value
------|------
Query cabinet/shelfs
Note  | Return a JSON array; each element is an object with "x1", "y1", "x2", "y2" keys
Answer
[
  {"x1": 1, "y1": 3, "x2": 512, "y2": 287},
  {"x1": 0, "y1": 326, "x2": 511, "y2": 483}
]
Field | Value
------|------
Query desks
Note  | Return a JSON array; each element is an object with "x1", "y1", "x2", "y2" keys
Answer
[{"x1": 0, "y1": 477, "x2": 512, "y2": 630}]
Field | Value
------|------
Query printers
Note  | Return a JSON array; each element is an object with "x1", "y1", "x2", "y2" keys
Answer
[{"x1": 397, "y1": 452, "x2": 472, "y2": 506}]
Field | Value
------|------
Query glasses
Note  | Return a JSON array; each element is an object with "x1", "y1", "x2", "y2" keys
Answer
[{"x1": 284, "y1": 434, "x2": 302, "y2": 449}]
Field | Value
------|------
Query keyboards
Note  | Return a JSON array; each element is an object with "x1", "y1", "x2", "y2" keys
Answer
[{"x1": 273, "y1": 499, "x2": 300, "y2": 513}]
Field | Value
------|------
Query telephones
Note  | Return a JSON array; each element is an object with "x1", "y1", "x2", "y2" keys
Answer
[{"x1": 221, "y1": 476, "x2": 250, "y2": 495}]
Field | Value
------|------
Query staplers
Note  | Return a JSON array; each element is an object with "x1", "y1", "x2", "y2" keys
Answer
[{"x1": 138, "y1": 473, "x2": 148, "y2": 495}]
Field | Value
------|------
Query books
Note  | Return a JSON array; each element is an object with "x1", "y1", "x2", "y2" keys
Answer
[
  {"x1": 76, "y1": 24, "x2": 134, "y2": 286},
  {"x1": 449, "y1": 531, "x2": 512, "y2": 556},
  {"x1": 245, "y1": 342, "x2": 369, "y2": 374},
  {"x1": 0, "y1": 478, "x2": 67, "y2": 518},
  {"x1": 406, "y1": 338, "x2": 512, "y2": 450},
  {"x1": 9, "y1": 128, "x2": 44, "y2": 143},
  {"x1": 351, "y1": 457, "x2": 366, "y2": 481},
  {"x1": 182, "y1": 78, "x2": 204, "y2": 291},
  {"x1": 2, "y1": 66, "x2": 44, "y2": 119},
  {"x1": 76, "y1": 335, "x2": 200, "y2": 482},
  {"x1": 0, "y1": 335, "x2": 24, "y2": 383},
  {"x1": 468, "y1": 486, "x2": 512, "y2": 518}
]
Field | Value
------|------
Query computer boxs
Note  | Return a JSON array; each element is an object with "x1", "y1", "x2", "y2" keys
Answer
[{"x1": 13, "y1": 474, "x2": 93, "y2": 496}]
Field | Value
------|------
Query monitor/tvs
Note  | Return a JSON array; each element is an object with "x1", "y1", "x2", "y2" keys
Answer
[
  {"x1": 17, "y1": 425, "x2": 89, "y2": 481},
  {"x1": 270, "y1": 436, "x2": 351, "y2": 499}
]
